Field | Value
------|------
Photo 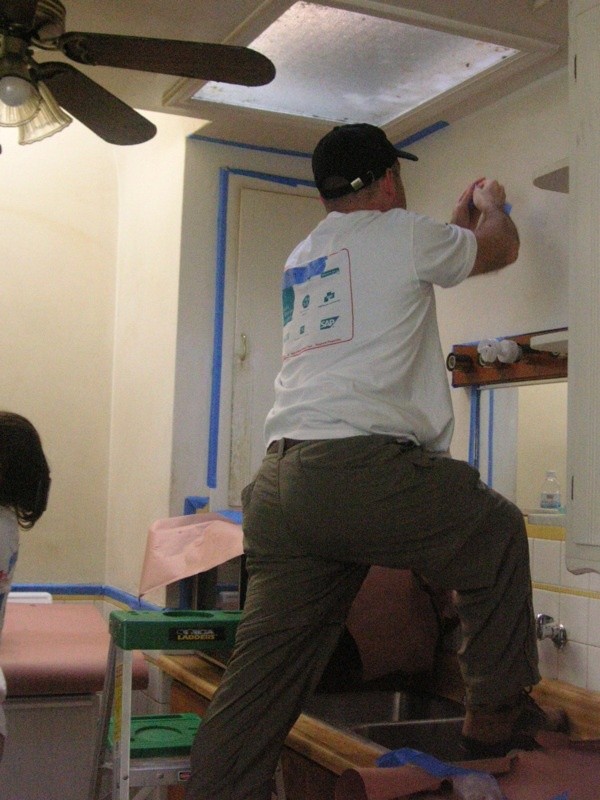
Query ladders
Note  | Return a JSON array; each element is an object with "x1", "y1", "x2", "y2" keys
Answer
[{"x1": 89, "y1": 610, "x2": 287, "y2": 800}]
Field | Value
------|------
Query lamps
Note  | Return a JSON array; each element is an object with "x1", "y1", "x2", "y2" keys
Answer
[{"x1": 0, "y1": 53, "x2": 72, "y2": 145}]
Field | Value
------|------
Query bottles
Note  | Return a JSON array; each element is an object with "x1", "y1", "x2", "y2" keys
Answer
[{"x1": 540, "y1": 471, "x2": 563, "y2": 516}]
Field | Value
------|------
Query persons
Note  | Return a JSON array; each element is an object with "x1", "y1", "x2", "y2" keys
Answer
[
  {"x1": 0, "y1": 410, "x2": 51, "y2": 634},
  {"x1": 190, "y1": 123, "x2": 570, "y2": 800}
]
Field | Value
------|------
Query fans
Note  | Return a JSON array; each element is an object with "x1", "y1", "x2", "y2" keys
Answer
[{"x1": 0, "y1": 0, "x2": 277, "y2": 146}]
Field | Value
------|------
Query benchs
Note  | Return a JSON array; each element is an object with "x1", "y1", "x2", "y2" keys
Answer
[{"x1": 0, "y1": 599, "x2": 147, "y2": 800}]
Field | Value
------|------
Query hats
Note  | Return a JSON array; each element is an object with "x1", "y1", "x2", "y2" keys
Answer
[{"x1": 311, "y1": 123, "x2": 420, "y2": 198}]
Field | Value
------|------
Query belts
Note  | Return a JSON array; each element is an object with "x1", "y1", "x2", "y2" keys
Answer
[{"x1": 265, "y1": 436, "x2": 302, "y2": 454}]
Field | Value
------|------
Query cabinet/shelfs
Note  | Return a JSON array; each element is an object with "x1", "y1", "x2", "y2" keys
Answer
[{"x1": 526, "y1": 151, "x2": 569, "y2": 526}]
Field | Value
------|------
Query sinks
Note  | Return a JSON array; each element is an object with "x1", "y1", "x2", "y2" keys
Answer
[
  {"x1": 301, "y1": 686, "x2": 467, "y2": 727},
  {"x1": 353, "y1": 716, "x2": 514, "y2": 763}
]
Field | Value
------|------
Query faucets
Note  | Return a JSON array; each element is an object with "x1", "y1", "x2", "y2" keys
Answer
[{"x1": 534, "y1": 612, "x2": 558, "y2": 640}]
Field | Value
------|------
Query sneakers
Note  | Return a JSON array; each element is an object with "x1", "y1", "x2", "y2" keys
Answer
[{"x1": 457, "y1": 687, "x2": 569, "y2": 759}]
